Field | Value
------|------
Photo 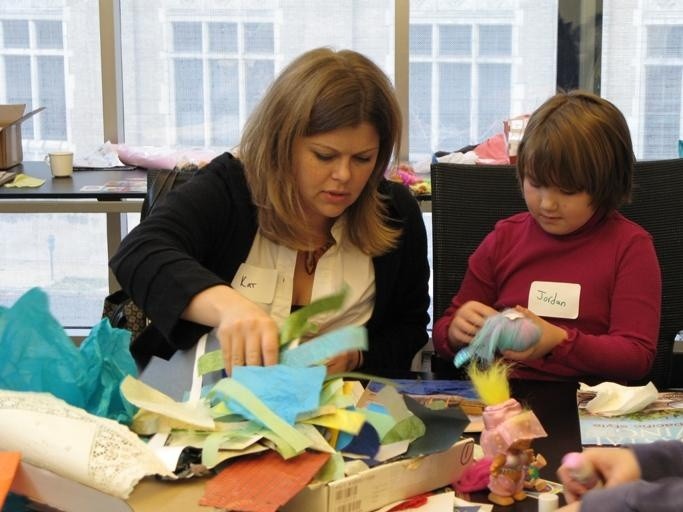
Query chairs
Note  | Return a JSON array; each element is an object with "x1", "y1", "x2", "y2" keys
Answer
[{"x1": 430, "y1": 157, "x2": 683, "y2": 381}]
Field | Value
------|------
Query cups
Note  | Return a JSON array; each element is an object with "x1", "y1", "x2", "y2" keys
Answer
[{"x1": 43, "y1": 151, "x2": 73, "y2": 178}]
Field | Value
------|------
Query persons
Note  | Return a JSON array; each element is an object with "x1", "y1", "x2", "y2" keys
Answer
[
  {"x1": 433, "y1": 91, "x2": 663, "y2": 382},
  {"x1": 552, "y1": 439, "x2": 683, "y2": 512},
  {"x1": 108, "y1": 47, "x2": 430, "y2": 389}
]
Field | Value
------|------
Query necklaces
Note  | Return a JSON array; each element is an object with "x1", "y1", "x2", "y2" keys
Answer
[{"x1": 304, "y1": 237, "x2": 335, "y2": 275}]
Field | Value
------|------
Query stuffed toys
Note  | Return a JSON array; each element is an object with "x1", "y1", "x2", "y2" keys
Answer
[
  {"x1": 524, "y1": 453, "x2": 547, "y2": 491},
  {"x1": 453, "y1": 308, "x2": 543, "y2": 368},
  {"x1": 457, "y1": 353, "x2": 547, "y2": 505}
]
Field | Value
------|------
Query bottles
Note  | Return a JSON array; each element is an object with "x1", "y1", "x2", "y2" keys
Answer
[{"x1": 561, "y1": 452, "x2": 603, "y2": 492}]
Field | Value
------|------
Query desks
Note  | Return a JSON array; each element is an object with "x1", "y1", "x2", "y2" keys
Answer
[{"x1": 0, "y1": 162, "x2": 431, "y2": 294}]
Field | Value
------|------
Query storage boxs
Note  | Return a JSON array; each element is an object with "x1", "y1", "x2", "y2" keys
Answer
[{"x1": 0, "y1": 102, "x2": 45, "y2": 168}]
